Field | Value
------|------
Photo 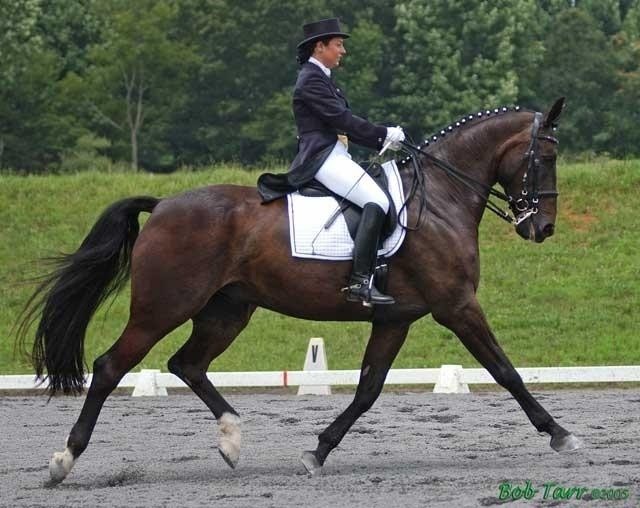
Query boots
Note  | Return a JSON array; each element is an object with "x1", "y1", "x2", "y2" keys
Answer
[{"x1": 344, "y1": 201, "x2": 396, "y2": 307}]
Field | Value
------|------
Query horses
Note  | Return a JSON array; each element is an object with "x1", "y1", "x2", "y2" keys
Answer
[{"x1": 2, "y1": 92, "x2": 585, "y2": 483}]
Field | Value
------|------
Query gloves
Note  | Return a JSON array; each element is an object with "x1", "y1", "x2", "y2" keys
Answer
[{"x1": 382, "y1": 126, "x2": 406, "y2": 152}]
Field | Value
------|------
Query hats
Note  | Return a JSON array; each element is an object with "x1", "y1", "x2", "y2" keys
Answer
[{"x1": 296, "y1": 18, "x2": 349, "y2": 50}]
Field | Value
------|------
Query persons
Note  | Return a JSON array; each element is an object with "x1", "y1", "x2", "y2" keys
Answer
[{"x1": 256, "y1": 16, "x2": 408, "y2": 305}]
